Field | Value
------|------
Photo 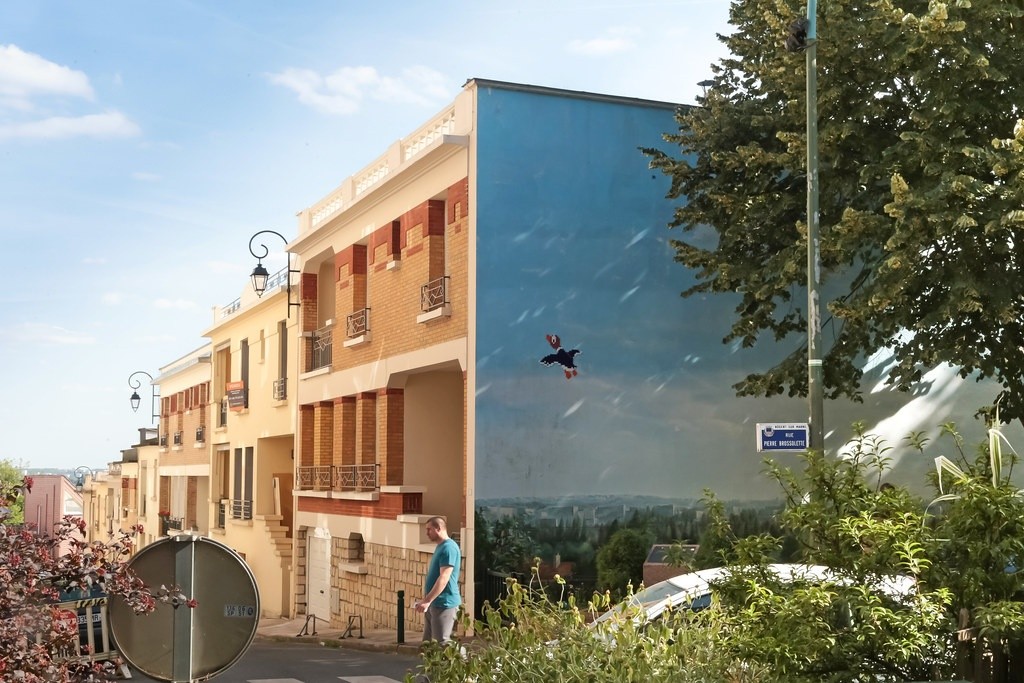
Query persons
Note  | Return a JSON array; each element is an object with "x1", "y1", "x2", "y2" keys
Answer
[{"x1": 415, "y1": 517, "x2": 469, "y2": 663}]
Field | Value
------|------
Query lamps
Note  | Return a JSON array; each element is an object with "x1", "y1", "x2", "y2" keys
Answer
[
  {"x1": 129, "y1": 371, "x2": 163, "y2": 423},
  {"x1": 74, "y1": 466, "x2": 93, "y2": 493},
  {"x1": 249, "y1": 231, "x2": 301, "y2": 319}
]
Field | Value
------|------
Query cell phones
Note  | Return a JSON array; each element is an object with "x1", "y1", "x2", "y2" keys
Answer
[{"x1": 414, "y1": 602, "x2": 427, "y2": 612}]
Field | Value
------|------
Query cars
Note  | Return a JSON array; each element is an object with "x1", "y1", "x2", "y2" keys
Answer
[{"x1": 452, "y1": 560, "x2": 948, "y2": 683}]
